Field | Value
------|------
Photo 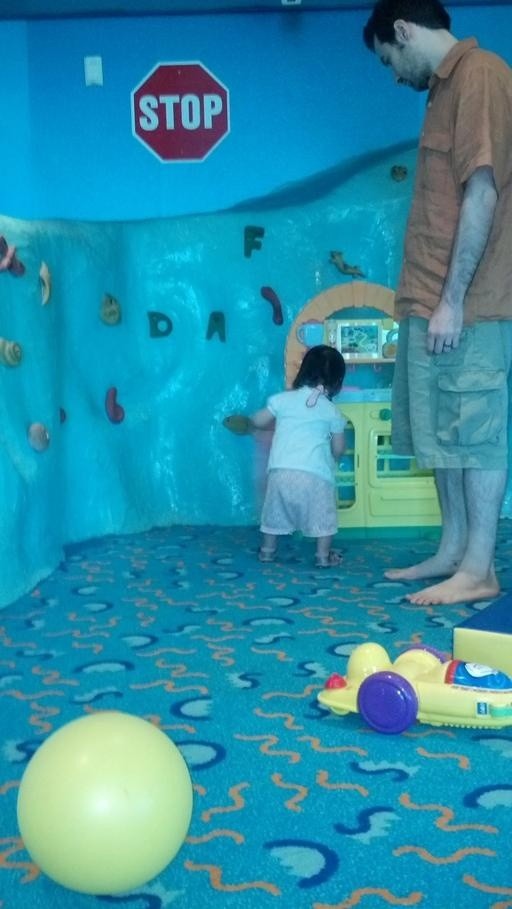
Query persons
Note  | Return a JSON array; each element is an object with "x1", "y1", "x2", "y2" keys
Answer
[
  {"x1": 251, "y1": 340, "x2": 347, "y2": 568},
  {"x1": 362, "y1": 0, "x2": 511, "y2": 606}
]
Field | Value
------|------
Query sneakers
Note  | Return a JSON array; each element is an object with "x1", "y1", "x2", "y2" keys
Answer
[
  {"x1": 258, "y1": 545, "x2": 278, "y2": 562},
  {"x1": 315, "y1": 548, "x2": 344, "y2": 568}
]
